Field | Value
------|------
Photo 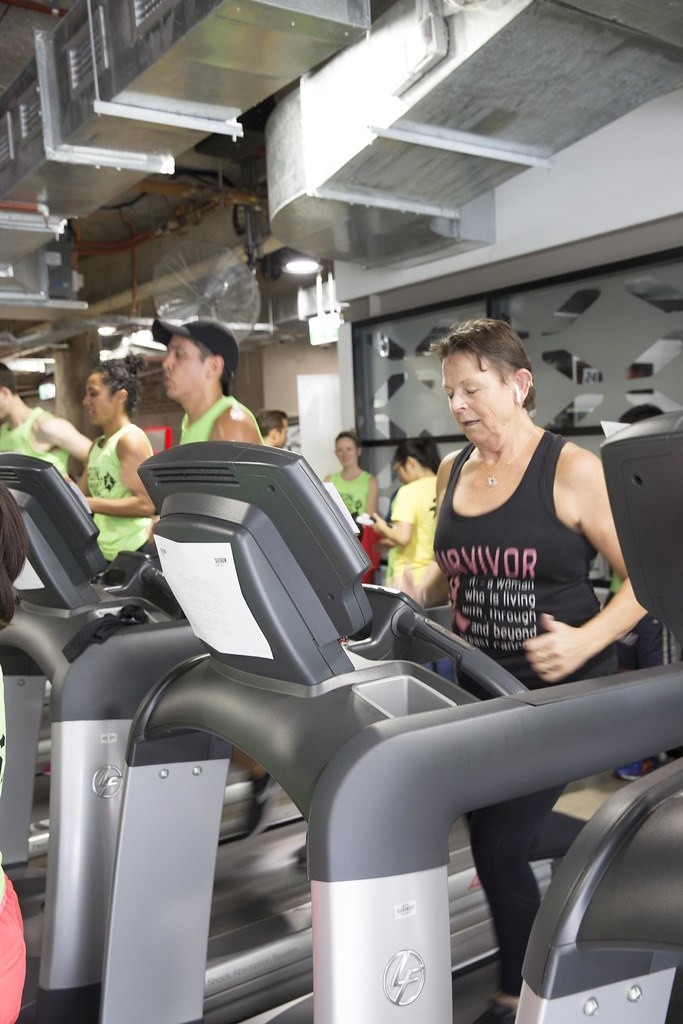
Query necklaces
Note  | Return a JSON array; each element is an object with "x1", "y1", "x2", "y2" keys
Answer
[{"x1": 479, "y1": 432, "x2": 534, "y2": 486}]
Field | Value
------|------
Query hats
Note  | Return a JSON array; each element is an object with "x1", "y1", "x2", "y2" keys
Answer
[{"x1": 151, "y1": 319, "x2": 237, "y2": 379}]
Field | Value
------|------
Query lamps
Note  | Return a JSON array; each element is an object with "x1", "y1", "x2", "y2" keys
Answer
[{"x1": 279, "y1": 246, "x2": 325, "y2": 276}]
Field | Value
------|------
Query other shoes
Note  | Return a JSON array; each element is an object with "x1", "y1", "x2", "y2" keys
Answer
[
  {"x1": 473, "y1": 999, "x2": 516, "y2": 1024},
  {"x1": 247, "y1": 775, "x2": 275, "y2": 831}
]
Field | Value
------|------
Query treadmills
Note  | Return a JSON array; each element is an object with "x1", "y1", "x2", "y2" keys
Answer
[{"x1": 1, "y1": 437, "x2": 683, "y2": 1024}]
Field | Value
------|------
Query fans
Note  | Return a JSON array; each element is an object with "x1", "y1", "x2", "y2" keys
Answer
[{"x1": 149, "y1": 236, "x2": 259, "y2": 343}]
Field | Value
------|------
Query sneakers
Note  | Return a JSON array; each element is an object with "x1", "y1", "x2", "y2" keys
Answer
[{"x1": 614, "y1": 756, "x2": 660, "y2": 780}]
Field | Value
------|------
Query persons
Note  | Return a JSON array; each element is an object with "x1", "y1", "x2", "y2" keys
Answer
[
  {"x1": 151, "y1": 318, "x2": 282, "y2": 841},
  {"x1": 0, "y1": 363, "x2": 95, "y2": 495},
  {"x1": 389, "y1": 318, "x2": 648, "y2": 1024},
  {"x1": 0, "y1": 481, "x2": 27, "y2": 1024},
  {"x1": 257, "y1": 411, "x2": 287, "y2": 448},
  {"x1": 373, "y1": 430, "x2": 457, "y2": 682},
  {"x1": 82, "y1": 353, "x2": 161, "y2": 569},
  {"x1": 323, "y1": 433, "x2": 378, "y2": 540},
  {"x1": 602, "y1": 404, "x2": 665, "y2": 782}
]
